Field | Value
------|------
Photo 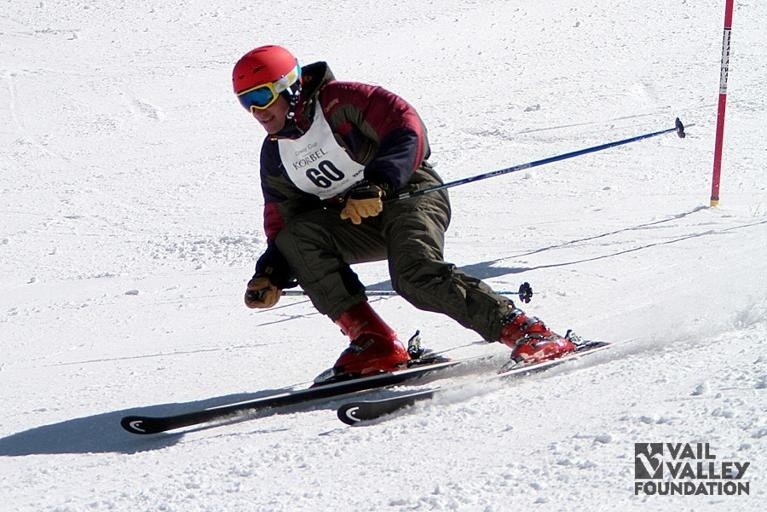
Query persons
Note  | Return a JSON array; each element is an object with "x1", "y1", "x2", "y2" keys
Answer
[{"x1": 232, "y1": 45, "x2": 577, "y2": 385}]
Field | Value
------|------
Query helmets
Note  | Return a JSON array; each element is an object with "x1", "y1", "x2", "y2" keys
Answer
[{"x1": 232, "y1": 45, "x2": 297, "y2": 93}]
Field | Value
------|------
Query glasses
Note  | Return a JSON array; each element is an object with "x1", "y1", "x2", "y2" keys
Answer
[{"x1": 236, "y1": 64, "x2": 302, "y2": 114}]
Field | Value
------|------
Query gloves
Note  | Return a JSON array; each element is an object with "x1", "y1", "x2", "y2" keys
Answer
[
  {"x1": 245, "y1": 274, "x2": 282, "y2": 308},
  {"x1": 340, "y1": 182, "x2": 386, "y2": 224}
]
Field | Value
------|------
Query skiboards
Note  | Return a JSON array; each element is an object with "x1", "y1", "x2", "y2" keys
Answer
[{"x1": 121, "y1": 341, "x2": 636, "y2": 436}]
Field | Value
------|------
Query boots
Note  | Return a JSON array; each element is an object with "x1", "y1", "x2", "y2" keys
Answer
[
  {"x1": 501, "y1": 307, "x2": 577, "y2": 366},
  {"x1": 333, "y1": 301, "x2": 410, "y2": 376}
]
